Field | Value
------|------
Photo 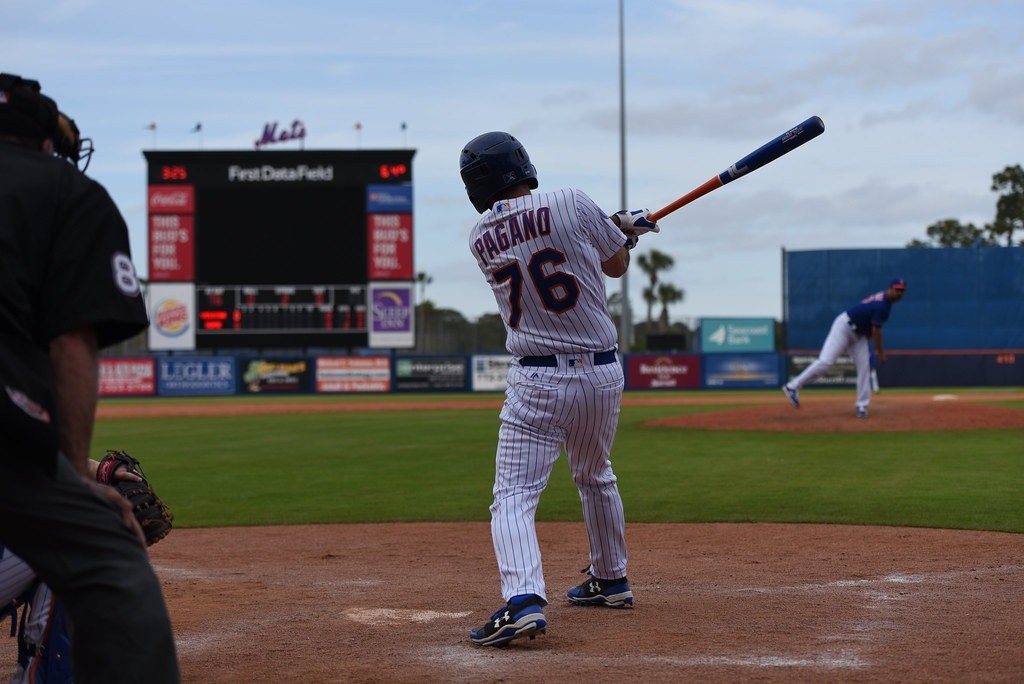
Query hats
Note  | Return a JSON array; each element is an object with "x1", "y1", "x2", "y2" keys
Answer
[{"x1": 890, "y1": 278, "x2": 906, "y2": 291}]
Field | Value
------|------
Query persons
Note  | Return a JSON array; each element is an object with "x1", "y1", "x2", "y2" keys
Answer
[
  {"x1": 780, "y1": 278, "x2": 907, "y2": 417},
  {"x1": 0, "y1": 71, "x2": 182, "y2": 682},
  {"x1": 459, "y1": 130, "x2": 660, "y2": 647}
]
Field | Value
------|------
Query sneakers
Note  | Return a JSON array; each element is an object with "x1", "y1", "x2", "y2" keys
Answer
[
  {"x1": 782, "y1": 385, "x2": 800, "y2": 410},
  {"x1": 566, "y1": 563, "x2": 634, "y2": 608},
  {"x1": 470, "y1": 594, "x2": 547, "y2": 648},
  {"x1": 856, "y1": 407, "x2": 869, "y2": 419}
]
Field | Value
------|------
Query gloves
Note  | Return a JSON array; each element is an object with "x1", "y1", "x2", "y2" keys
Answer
[{"x1": 612, "y1": 208, "x2": 660, "y2": 251}]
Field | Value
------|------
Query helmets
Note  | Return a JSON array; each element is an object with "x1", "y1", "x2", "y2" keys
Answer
[
  {"x1": 460, "y1": 132, "x2": 538, "y2": 214},
  {"x1": 0, "y1": 73, "x2": 58, "y2": 148}
]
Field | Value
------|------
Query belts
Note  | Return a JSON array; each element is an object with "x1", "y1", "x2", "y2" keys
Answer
[
  {"x1": 519, "y1": 348, "x2": 618, "y2": 367},
  {"x1": 848, "y1": 321, "x2": 862, "y2": 336}
]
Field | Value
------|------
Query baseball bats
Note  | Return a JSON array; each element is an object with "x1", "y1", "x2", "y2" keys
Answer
[{"x1": 646, "y1": 115, "x2": 825, "y2": 223}]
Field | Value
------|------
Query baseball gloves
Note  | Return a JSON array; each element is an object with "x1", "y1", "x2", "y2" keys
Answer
[{"x1": 94, "y1": 448, "x2": 175, "y2": 549}]
